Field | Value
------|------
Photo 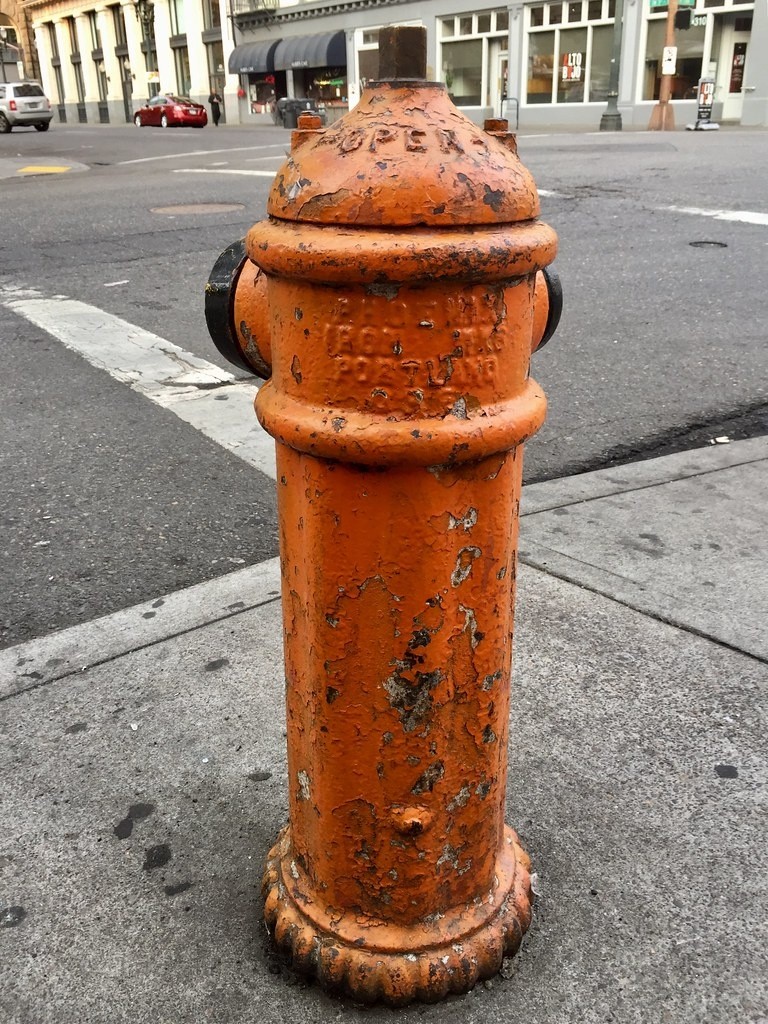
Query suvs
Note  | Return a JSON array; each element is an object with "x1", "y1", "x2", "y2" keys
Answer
[{"x1": 0, "y1": 82, "x2": 53, "y2": 135}]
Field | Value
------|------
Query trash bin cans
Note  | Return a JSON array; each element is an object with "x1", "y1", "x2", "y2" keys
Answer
[
  {"x1": 297, "y1": 99, "x2": 315, "y2": 118},
  {"x1": 281, "y1": 100, "x2": 300, "y2": 128}
]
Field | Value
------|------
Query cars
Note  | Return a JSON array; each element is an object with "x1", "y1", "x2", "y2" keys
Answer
[
  {"x1": 251, "y1": 92, "x2": 276, "y2": 114},
  {"x1": 132, "y1": 94, "x2": 208, "y2": 130}
]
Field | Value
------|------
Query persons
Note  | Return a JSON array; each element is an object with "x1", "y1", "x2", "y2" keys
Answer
[{"x1": 208, "y1": 88, "x2": 222, "y2": 127}]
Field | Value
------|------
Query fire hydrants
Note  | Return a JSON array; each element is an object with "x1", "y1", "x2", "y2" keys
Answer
[{"x1": 204, "y1": 24, "x2": 560, "y2": 1003}]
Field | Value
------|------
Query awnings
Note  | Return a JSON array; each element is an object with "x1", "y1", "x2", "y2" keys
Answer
[
  {"x1": 229, "y1": 38, "x2": 282, "y2": 74},
  {"x1": 274, "y1": 29, "x2": 346, "y2": 71}
]
